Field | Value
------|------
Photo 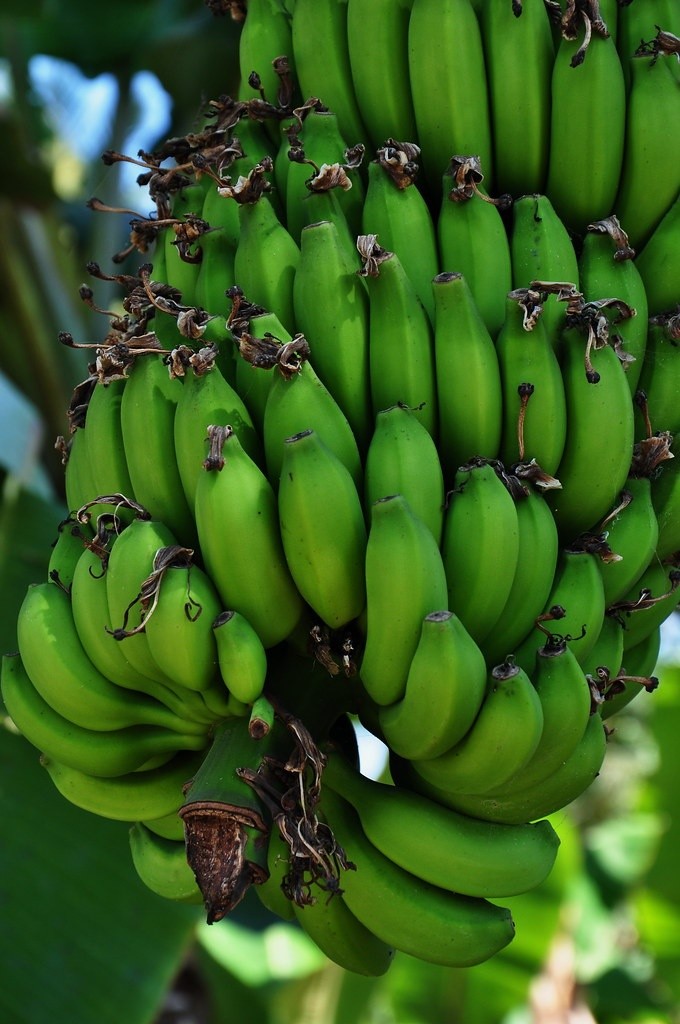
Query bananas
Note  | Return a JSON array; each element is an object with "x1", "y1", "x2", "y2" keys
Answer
[{"x1": 0, "y1": 0, "x2": 680, "y2": 977}]
